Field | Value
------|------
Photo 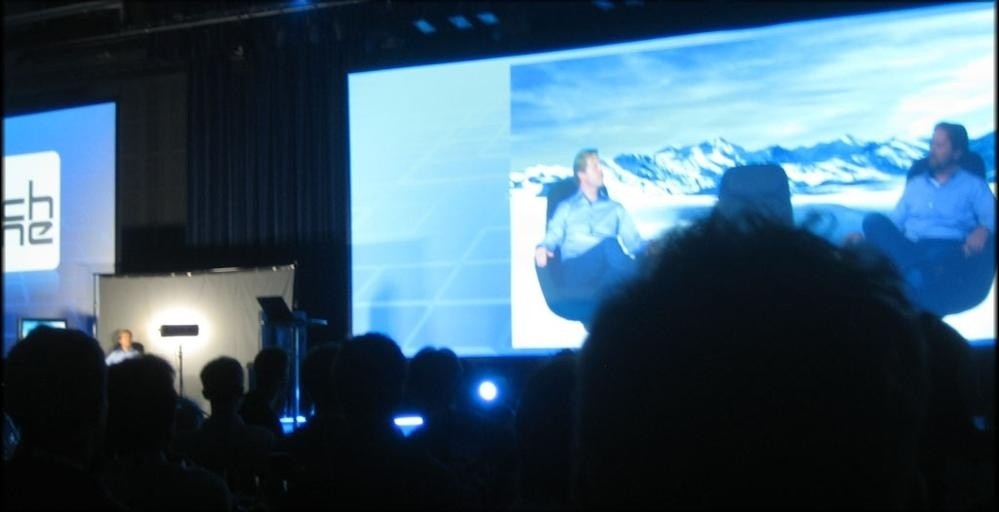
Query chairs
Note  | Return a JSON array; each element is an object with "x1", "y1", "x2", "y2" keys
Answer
[
  {"x1": 532, "y1": 178, "x2": 621, "y2": 337},
  {"x1": 901, "y1": 154, "x2": 995, "y2": 318}
]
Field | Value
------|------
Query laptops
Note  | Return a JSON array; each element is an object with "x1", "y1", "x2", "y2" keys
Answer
[{"x1": 255, "y1": 295, "x2": 293, "y2": 320}]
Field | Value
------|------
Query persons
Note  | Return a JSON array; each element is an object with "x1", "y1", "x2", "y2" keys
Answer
[
  {"x1": 0, "y1": 325, "x2": 575, "y2": 512},
  {"x1": 580, "y1": 212, "x2": 999, "y2": 512},
  {"x1": 844, "y1": 121, "x2": 997, "y2": 306},
  {"x1": 532, "y1": 148, "x2": 642, "y2": 336}
]
